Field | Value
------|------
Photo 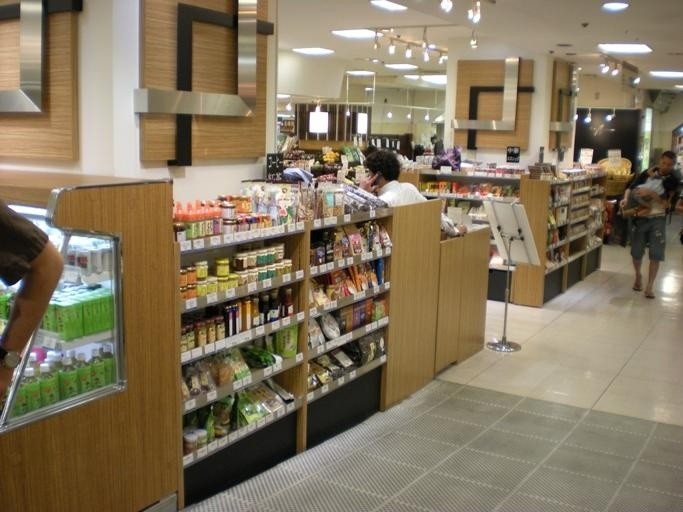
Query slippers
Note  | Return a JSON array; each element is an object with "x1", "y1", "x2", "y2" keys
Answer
[
  {"x1": 632, "y1": 273, "x2": 643, "y2": 291},
  {"x1": 645, "y1": 290, "x2": 655, "y2": 299}
]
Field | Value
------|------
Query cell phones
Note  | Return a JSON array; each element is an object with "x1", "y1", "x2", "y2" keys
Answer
[{"x1": 373, "y1": 170, "x2": 383, "y2": 185}]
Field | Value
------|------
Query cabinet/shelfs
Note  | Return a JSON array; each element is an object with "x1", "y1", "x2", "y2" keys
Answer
[
  {"x1": 170, "y1": 212, "x2": 389, "y2": 507},
  {"x1": 405, "y1": 159, "x2": 607, "y2": 310}
]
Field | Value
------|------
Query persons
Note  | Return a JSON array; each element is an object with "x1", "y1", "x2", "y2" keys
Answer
[
  {"x1": 359, "y1": 150, "x2": 467, "y2": 237},
  {"x1": 0, "y1": 198, "x2": 64, "y2": 404},
  {"x1": 366, "y1": 145, "x2": 377, "y2": 157},
  {"x1": 618, "y1": 151, "x2": 679, "y2": 297},
  {"x1": 431, "y1": 135, "x2": 442, "y2": 155},
  {"x1": 631, "y1": 172, "x2": 676, "y2": 219}
]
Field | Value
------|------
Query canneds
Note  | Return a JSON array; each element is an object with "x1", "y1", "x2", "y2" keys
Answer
[
  {"x1": 201, "y1": 194, "x2": 272, "y2": 235},
  {"x1": 179, "y1": 239, "x2": 293, "y2": 301},
  {"x1": 173, "y1": 222, "x2": 186, "y2": 242},
  {"x1": 181, "y1": 314, "x2": 226, "y2": 355}
]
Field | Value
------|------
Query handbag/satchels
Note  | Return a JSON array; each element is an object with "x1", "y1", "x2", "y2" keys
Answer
[{"x1": 619, "y1": 178, "x2": 667, "y2": 218}]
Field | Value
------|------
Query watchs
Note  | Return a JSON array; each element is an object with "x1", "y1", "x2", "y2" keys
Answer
[{"x1": 0, "y1": 348, "x2": 22, "y2": 369}]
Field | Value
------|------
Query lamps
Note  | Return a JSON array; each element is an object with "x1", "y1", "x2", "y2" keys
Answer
[
  {"x1": 369, "y1": 0, "x2": 486, "y2": 66},
  {"x1": 284, "y1": 100, "x2": 431, "y2": 122},
  {"x1": 584, "y1": 104, "x2": 618, "y2": 126},
  {"x1": 600, "y1": 56, "x2": 623, "y2": 77}
]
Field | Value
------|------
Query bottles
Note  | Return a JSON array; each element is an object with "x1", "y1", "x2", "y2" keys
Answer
[
  {"x1": 222, "y1": 287, "x2": 295, "y2": 337},
  {"x1": 6, "y1": 345, "x2": 115, "y2": 421},
  {"x1": 173, "y1": 197, "x2": 224, "y2": 238}
]
